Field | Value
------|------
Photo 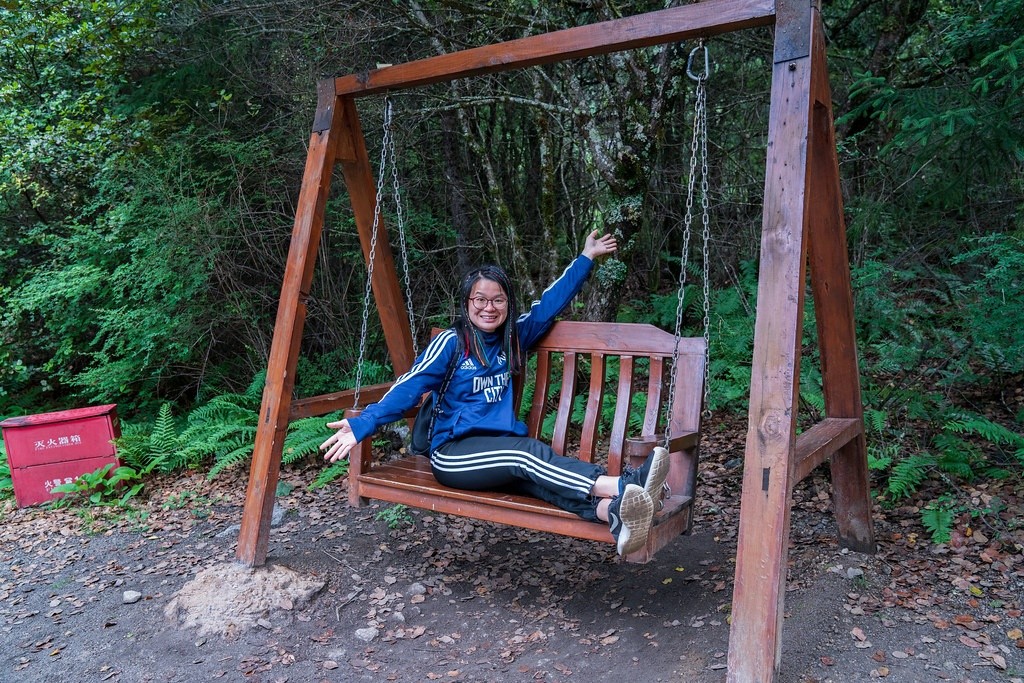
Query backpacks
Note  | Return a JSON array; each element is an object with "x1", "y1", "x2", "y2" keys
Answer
[{"x1": 410, "y1": 327, "x2": 461, "y2": 458}]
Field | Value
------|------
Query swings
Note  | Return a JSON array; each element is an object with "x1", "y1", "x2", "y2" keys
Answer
[{"x1": 347, "y1": 36, "x2": 711, "y2": 564}]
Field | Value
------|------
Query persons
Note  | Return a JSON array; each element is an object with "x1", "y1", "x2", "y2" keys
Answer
[{"x1": 319, "y1": 230, "x2": 670, "y2": 556}]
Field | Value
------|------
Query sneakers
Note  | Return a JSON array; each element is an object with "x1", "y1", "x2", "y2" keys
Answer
[
  {"x1": 621, "y1": 446, "x2": 670, "y2": 515},
  {"x1": 607, "y1": 484, "x2": 654, "y2": 556}
]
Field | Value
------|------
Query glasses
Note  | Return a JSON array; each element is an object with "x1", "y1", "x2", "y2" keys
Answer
[{"x1": 469, "y1": 296, "x2": 508, "y2": 310}]
